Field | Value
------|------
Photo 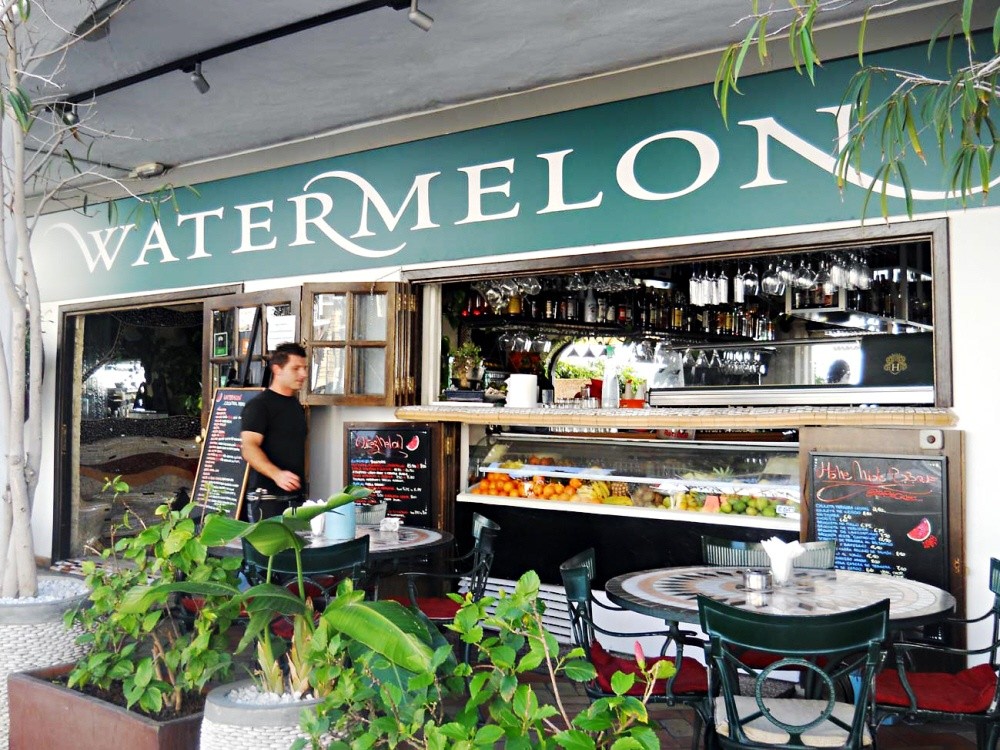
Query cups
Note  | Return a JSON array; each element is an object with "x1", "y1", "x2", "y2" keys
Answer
[
  {"x1": 542, "y1": 390, "x2": 553, "y2": 408},
  {"x1": 557, "y1": 386, "x2": 599, "y2": 409}
]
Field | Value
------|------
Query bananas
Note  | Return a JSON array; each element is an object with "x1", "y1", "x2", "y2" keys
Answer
[{"x1": 574, "y1": 481, "x2": 632, "y2": 505}]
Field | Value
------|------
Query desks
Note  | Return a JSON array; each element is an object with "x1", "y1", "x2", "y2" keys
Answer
[
  {"x1": 606, "y1": 566, "x2": 957, "y2": 703},
  {"x1": 210, "y1": 517, "x2": 455, "y2": 609}
]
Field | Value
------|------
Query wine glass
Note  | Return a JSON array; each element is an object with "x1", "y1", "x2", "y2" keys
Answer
[
  {"x1": 565, "y1": 342, "x2": 766, "y2": 375},
  {"x1": 568, "y1": 269, "x2": 640, "y2": 293},
  {"x1": 498, "y1": 330, "x2": 551, "y2": 354},
  {"x1": 689, "y1": 249, "x2": 872, "y2": 306}
]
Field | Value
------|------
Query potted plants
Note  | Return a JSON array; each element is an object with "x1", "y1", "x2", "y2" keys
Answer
[
  {"x1": 125, "y1": 483, "x2": 464, "y2": 750},
  {"x1": 552, "y1": 358, "x2": 646, "y2": 409},
  {"x1": 448, "y1": 342, "x2": 487, "y2": 391},
  {"x1": 8, "y1": 475, "x2": 281, "y2": 750}
]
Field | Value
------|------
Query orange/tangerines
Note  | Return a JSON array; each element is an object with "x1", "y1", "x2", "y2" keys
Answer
[
  {"x1": 658, "y1": 494, "x2": 787, "y2": 516},
  {"x1": 475, "y1": 457, "x2": 581, "y2": 501}
]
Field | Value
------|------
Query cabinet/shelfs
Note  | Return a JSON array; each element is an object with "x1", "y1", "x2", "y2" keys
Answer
[{"x1": 448, "y1": 276, "x2": 777, "y2": 384}]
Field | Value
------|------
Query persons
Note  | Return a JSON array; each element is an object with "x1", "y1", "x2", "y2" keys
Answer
[{"x1": 239, "y1": 343, "x2": 310, "y2": 523}]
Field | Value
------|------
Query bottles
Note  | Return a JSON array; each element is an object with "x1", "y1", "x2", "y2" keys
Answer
[
  {"x1": 466, "y1": 282, "x2": 776, "y2": 342},
  {"x1": 601, "y1": 345, "x2": 620, "y2": 409}
]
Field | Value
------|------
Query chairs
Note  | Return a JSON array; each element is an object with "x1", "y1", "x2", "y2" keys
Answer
[{"x1": 183, "y1": 512, "x2": 1000, "y2": 750}]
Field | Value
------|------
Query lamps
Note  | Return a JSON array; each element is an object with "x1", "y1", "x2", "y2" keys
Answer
[
  {"x1": 409, "y1": 0, "x2": 433, "y2": 31},
  {"x1": 190, "y1": 62, "x2": 210, "y2": 95},
  {"x1": 65, "y1": 103, "x2": 80, "y2": 124}
]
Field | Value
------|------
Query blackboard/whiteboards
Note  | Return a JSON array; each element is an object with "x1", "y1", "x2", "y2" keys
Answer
[
  {"x1": 190, "y1": 388, "x2": 265, "y2": 520},
  {"x1": 809, "y1": 452, "x2": 952, "y2": 644},
  {"x1": 342, "y1": 420, "x2": 448, "y2": 532}
]
